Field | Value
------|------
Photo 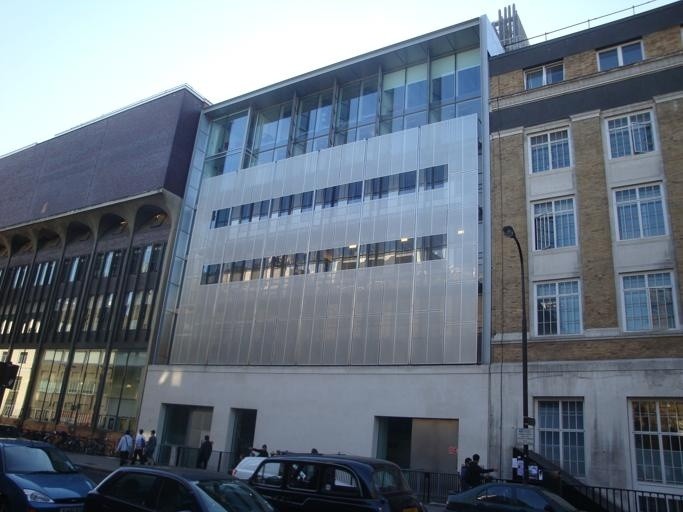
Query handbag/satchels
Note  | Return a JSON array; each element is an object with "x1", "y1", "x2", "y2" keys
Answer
[{"x1": 140, "y1": 439, "x2": 144, "y2": 448}]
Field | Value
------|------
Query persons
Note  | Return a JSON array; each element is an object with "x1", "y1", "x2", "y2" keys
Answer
[
  {"x1": 252, "y1": 444, "x2": 268, "y2": 457},
  {"x1": 196, "y1": 435, "x2": 212, "y2": 470},
  {"x1": 130, "y1": 429, "x2": 146, "y2": 465},
  {"x1": 114, "y1": 430, "x2": 134, "y2": 467},
  {"x1": 142, "y1": 429, "x2": 157, "y2": 466},
  {"x1": 465, "y1": 454, "x2": 495, "y2": 490},
  {"x1": 461, "y1": 457, "x2": 471, "y2": 483}
]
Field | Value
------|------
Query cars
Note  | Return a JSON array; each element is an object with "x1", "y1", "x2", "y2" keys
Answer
[
  {"x1": 85, "y1": 464, "x2": 273, "y2": 511},
  {"x1": 444, "y1": 483, "x2": 577, "y2": 511},
  {"x1": 0, "y1": 436, "x2": 97, "y2": 512},
  {"x1": 231, "y1": 455, "x2": 353, "y2": 486},
  {"x1": 246, "y1": 455, "x2": 426, "y2": 511}
]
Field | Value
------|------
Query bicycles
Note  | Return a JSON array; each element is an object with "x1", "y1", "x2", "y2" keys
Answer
[{"x1": 21, "y1": 427, "x2": 115, "y2": 456}]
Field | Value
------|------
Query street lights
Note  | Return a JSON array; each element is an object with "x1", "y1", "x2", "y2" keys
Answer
[{"x1": 501, "y1": 224, "x2": 531, "y2": 482}]
